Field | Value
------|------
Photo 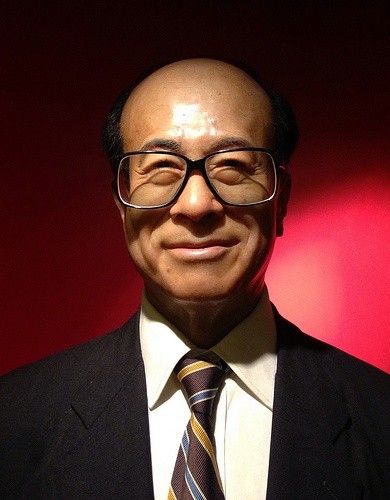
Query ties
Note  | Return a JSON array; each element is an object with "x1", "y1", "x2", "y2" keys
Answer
[{"x1": 167, "y1": 350, "x2": 234, "y2": 500}]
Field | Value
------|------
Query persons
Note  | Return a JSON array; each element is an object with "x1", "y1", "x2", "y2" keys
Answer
[{"x1": 1, "y1": 57, "x2": 390, "y2": 500}]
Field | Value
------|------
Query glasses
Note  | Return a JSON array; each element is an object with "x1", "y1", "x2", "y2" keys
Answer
[{"x1": 116, "y1": 147, "x2": 280, "y2": 209}]
